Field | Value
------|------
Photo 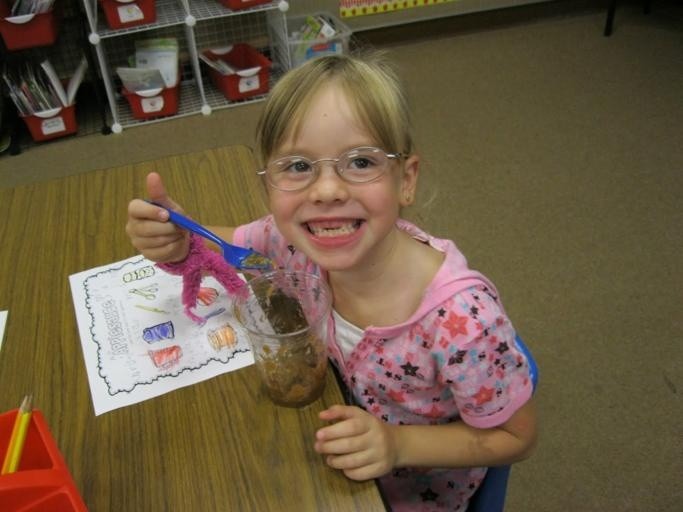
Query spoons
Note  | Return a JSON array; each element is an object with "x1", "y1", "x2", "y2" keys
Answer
[{"x1": 144, "y1": 199, "x2": 269, "y2": 270}]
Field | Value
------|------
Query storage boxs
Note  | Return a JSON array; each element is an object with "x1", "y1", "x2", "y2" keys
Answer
[
  {"x1": 217, "y1": 0, "x2": 273, "y2": 9},
  {"x1": 121, "y1": 84, "x2": 181, "y2": 120},
  {"x1": 285, "y1": 14, "x2": 355, "y2": 67},
  {"x1": 21, "y1": 91, "x2": 79, "y2": 138},
  {"x1": 199, "y1": 44, "x2": 273, "y2": 103},
  {"x1": 2, "y1": 7, "x2": 57, "y2": 48},
  {"x1": 100, "y1": 2, "x2": 156, "y2": 29}
]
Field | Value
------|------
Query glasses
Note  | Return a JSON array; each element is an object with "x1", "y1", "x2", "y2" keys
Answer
[{"x1": 255, "y1": 147, "x2": 413, "y2": 192}]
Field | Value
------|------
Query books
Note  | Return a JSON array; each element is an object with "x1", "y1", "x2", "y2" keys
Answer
[
  {"x1": 199, "y1": 52, "x2": 245, "y2": 75},
  {"x1": 114, "y1": 36, "x2": 178, "y2": 90},
  {"x1": 292, "y1": 14, "x2": 335, "y2": 56},
  {"x1": 2, "y1": 58, "x2": 68, "y2": 115}
]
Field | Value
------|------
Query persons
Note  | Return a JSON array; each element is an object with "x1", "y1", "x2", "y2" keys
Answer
[{"x1": 124, "y1": 53, "x2": 540, "y2": 512}]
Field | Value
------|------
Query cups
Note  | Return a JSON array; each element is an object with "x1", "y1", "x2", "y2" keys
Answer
[{"x1": 229, "y1": 270, "x2": 333, "y2": 409}]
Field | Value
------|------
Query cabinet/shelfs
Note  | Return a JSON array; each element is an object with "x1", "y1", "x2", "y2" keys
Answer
[{"x1": 83, "y1": 1, "x2": 288, "y2": 135}]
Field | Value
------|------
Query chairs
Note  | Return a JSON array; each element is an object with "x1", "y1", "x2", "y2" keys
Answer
[{"x1": 471, "y1": 336, "x2": 539, "y2": 512}]
